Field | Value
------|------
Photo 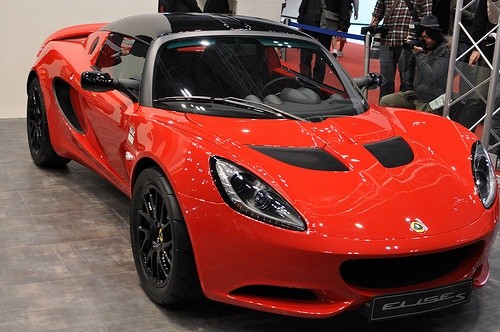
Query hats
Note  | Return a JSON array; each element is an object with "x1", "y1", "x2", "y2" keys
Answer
[{"x1": 414, "y1": 15, "x2": 444, "y2": 33}]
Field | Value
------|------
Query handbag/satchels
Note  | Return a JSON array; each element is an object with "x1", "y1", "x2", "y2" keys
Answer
[{"x1": 320, "y1": 10, "x2": 340, "y2": 31}]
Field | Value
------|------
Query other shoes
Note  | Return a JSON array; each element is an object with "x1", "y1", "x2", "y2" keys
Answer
[
  {"x1": 338, "y1": 50, "x2": 344, "y2": 57},
  {"x1": 332, "y1": 49, "x2": 338, "y2": 58}
]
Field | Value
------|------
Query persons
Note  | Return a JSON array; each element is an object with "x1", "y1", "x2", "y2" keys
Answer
[
  {"x1": 364, "y1": 0, "x2": 432, "y2": 105},
  {"x1": 159, "y1": 0, "x2": 236, "y2": 15},
  {"x1": 331, "y1": 0, "x2": 359, "y2": 58},
  {"x1": 449, "y1": 0, "x2": 500, "y2": 155},
  {"x1": 297, "y1": 0, "x2": 343, "y2": 84},
  {"x1": 379, "y1": 15, "x2": 451, "y2": 112}
]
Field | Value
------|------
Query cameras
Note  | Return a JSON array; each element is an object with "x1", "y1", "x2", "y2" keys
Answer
[{"x1": 402, "y1": 22, "x2": 426, "y2": 50}]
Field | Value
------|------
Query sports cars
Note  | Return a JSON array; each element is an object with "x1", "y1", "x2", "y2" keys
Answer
[{"x1": 26, "y1": 11, "x2": 500, "y2": 326}]
